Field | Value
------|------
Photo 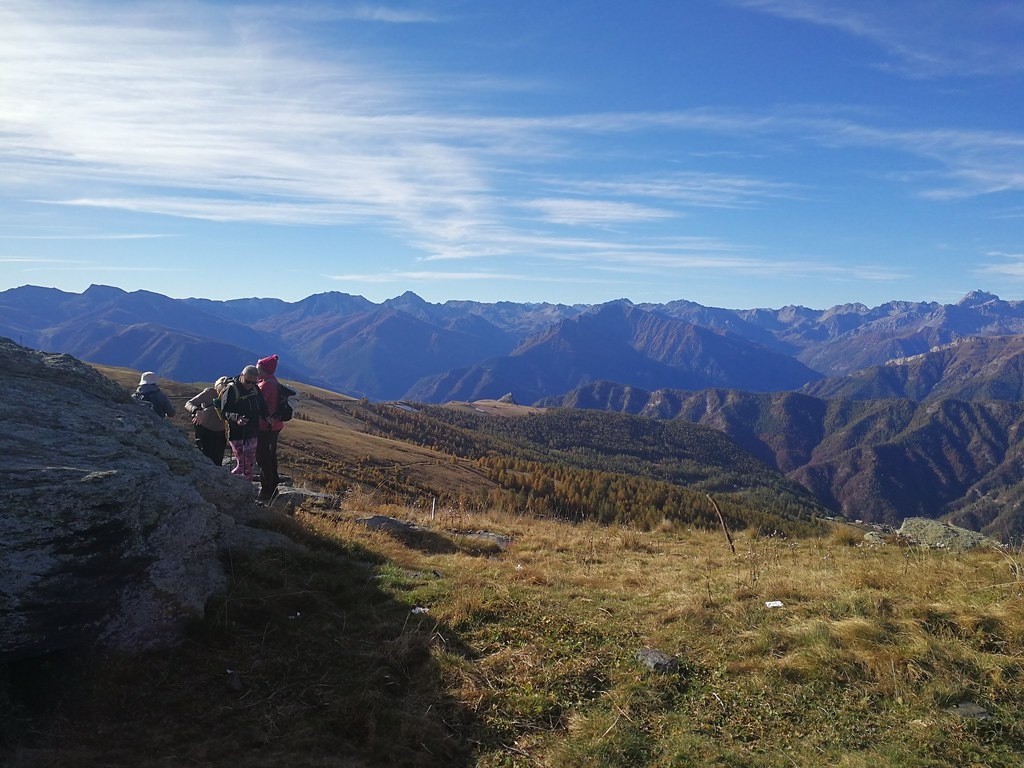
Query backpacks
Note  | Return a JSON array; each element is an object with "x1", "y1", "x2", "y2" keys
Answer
[{"x1": 266, "y1": 379, "x2": 300, "y2": 421}]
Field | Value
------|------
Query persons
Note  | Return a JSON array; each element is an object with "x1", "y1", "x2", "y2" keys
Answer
[
  {"x1": 132, "y1": 371, "x2": 177, "y2": 419},
  {"x1": 184, "y1": 376, "x2": 233, "y2": 466},
  {"x1": 253, "y1": 355, "x2": 285, "y2": 501},
  {"x1": 221, "y1": 365, "x2": 271, "y2": 483}
]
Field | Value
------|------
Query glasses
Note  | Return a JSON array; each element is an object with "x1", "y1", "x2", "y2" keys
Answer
[{"x1": 246, "y1": 379, "x2": 257, "y2": 385}]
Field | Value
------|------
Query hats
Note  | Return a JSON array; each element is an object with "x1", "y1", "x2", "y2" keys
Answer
[
  {"x1": 139, "y1": 372, "x2": 159, "y2": 385},
  {"x1": 257, "y1": 355, "x2": 278, "y2": 373},
  {"x1": 214, "y1": 376, "x2": 228, "y2": 387}
]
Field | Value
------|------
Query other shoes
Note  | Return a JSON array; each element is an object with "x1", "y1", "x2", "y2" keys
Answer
[{"x1": 257, "y1": 490, "x2": 278, "y2": 500}]
